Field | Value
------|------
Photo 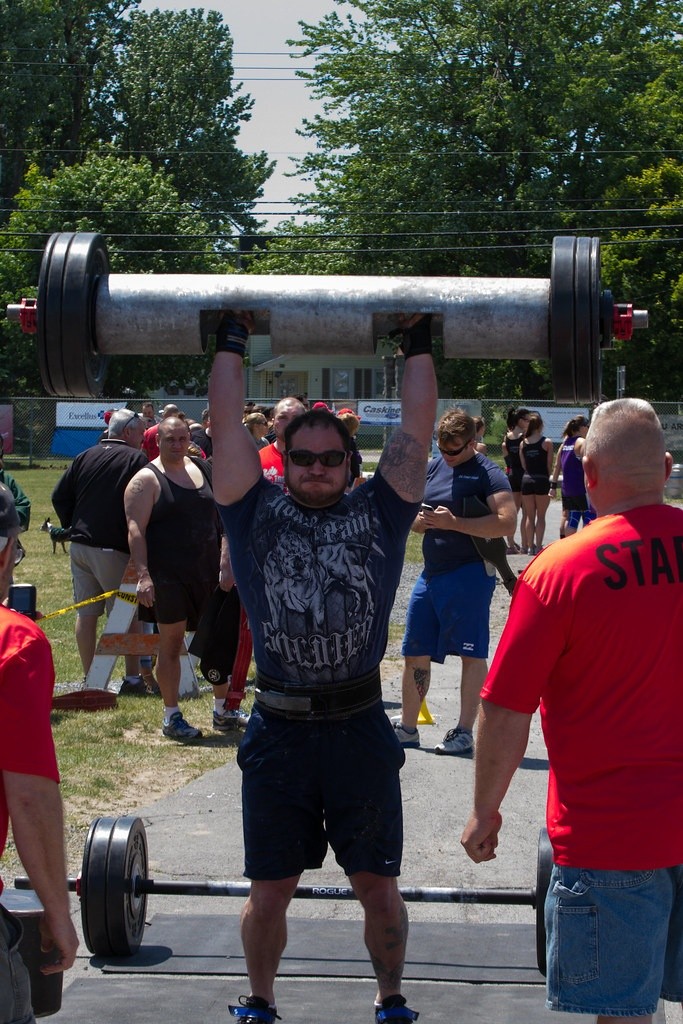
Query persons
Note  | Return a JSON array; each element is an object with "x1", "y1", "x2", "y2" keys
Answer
[
  {"x1": 501, "y1": 407, "x2": 597, "y2": 555},
  {"x1": 0, "y1": 480, "x2": 79, "y2": 1024},
  {"x1": 461, "y1": 397, "x2": 683, "y2": 1023},
  {"x1": 389, "y1": 411, "x2": 517, "y2": 755},
  {"x1": 123, "y1": 416, "x2": 235, "y2": 742},
  {"x1": 52, "y1": 395, "x2": 363, "y2": 696},
  {"x1": 0, "y1": 435, "x2": 30, "y2": 587},
  {"x1": 201, "y1": 310, "x2": 438, "y2": 1024}
]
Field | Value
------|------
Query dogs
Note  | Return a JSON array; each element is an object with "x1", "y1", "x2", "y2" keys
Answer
[{"x1": 39, "y1": 517, "x2": 72, "y2": 554}]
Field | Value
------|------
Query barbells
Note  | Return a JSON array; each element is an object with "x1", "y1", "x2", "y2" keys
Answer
[
  {"x1": 6, "y1": 232, "x2": 653, "y2": 409},
  {"x1": 12, "y1": 813, "x2": 555, "y2": 979}
]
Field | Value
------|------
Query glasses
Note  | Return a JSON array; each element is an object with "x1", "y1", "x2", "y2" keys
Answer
[
  {"x1": 435, "y1": 440, "x2": 471, "y2": 455},
  {"x1": 122, "y1": 413, "x2": 139, "y2": 434},
  {"x1": 15, "y1": 547, "x2": 26, "y2": 565},
  {"x1": 285, "y1": 449, "x2": 347, "y2": 467}
]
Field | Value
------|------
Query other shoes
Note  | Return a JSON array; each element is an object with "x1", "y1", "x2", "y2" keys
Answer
[
  {"x1": 117, "y1": 674, "x2": 146, "y2": 700},
  {"x1": 507, "y1": 542, "x2": 541, "y2": 557}
]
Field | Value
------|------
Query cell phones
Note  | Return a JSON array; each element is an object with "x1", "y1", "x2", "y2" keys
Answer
[
  {"x1": 421, "y1": 503, "x2": 434, "y2": 515},
  {"x1": 8, "y1": 583, "x2": 37, "y2": 623}
]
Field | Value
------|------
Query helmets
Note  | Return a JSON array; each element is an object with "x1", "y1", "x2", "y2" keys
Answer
[{"x1": 0, "y1": 482, "x2": 20, "y2": 538}]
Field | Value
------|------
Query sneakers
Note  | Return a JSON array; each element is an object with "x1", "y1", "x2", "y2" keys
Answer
[
  {"x1": 229, "y1": 993, "x2": 282, "y2": 1024},
  {"x1": 211, "y1": 709, "x2": 250, "y2": 730},
  {"x1": 374, "y1": 994, "x2": 420, "y2": 1024},
  {"x1": 433, "y1": 729, "x2": 474, "y2": 754},
  {"x1": 391, "y1": 724, "x2": 419, "y2": 747},
  {"x1": 163, "y1": 712, "x2": 201, "y2": 739}
]
